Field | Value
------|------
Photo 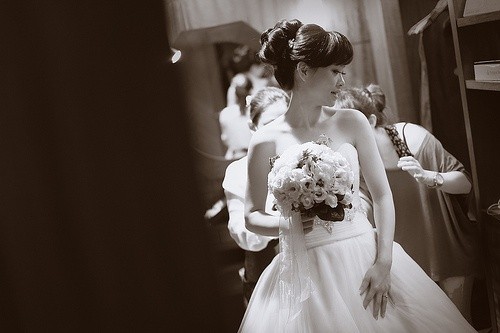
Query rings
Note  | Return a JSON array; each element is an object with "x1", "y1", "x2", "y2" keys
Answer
[{"x1": 383, "y1": 296, "x2": 389, "y2": 298}]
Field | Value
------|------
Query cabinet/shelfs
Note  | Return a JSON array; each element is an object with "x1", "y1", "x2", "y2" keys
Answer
[{"x1": 446, "y1": 0, "x2": 500, "y2": 269}]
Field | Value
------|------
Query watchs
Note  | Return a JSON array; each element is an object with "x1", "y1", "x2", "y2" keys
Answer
[{"x1": 426, "y1": 172, "x2": 444, "y2": 189}]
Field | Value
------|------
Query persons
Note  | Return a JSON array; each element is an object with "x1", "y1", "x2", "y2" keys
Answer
[
  {"x1": 222, "y1": 87, "x2": 292, "y2": 303},
  {"x1": 244, "y1": 18, "x2": 474, "y2": 333},
  {"x1": 219, "y1": 45, "x2": 286, "y2": 163}
]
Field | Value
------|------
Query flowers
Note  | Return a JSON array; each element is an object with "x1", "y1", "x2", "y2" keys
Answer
[{"x1": 267, "y1": 141, "x2": 354, "y2": 223}]
